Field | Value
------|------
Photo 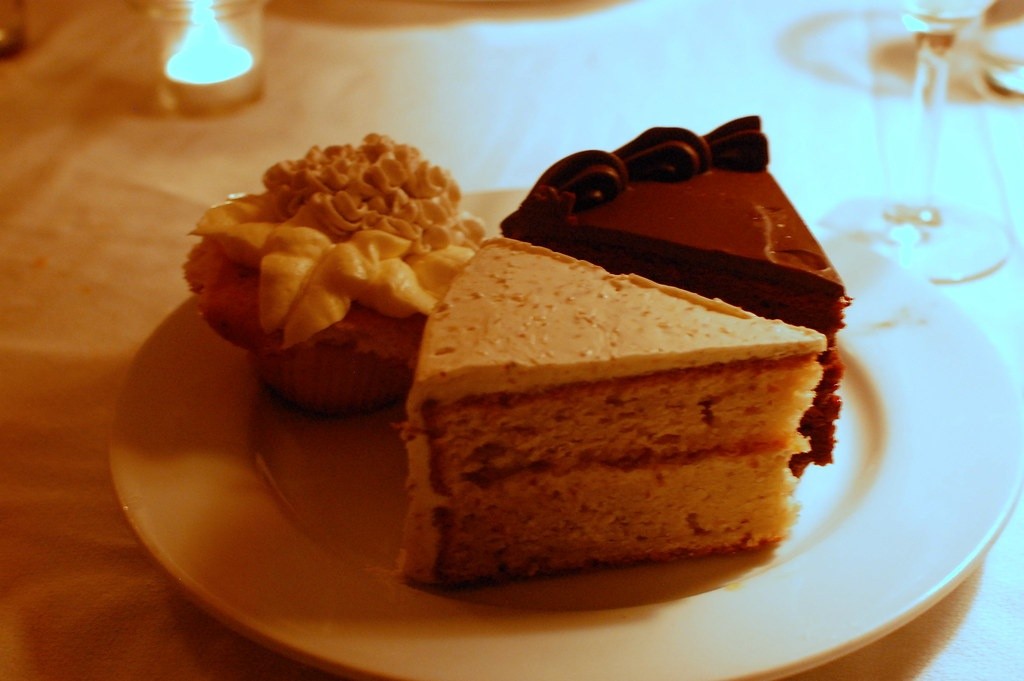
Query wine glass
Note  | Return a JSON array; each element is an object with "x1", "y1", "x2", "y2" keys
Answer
[{"x1": 819, "y1": 0, "x2": 1017, "y2": 284}]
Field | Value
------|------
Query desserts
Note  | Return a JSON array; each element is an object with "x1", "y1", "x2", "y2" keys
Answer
[
  {"x1": 178, "y1": 133, "x2": 488, "y2": 416},
  {"x1": 497, "y1": 115, "x2": 854, "y2": 480},
  {"x1": 393, "y1": 237, "x2": 827, "y2": 589}
]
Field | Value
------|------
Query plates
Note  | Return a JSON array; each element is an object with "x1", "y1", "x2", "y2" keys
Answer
[{"x1": 109, "y1": 189, "x2": 1024, "y2": 680}]
